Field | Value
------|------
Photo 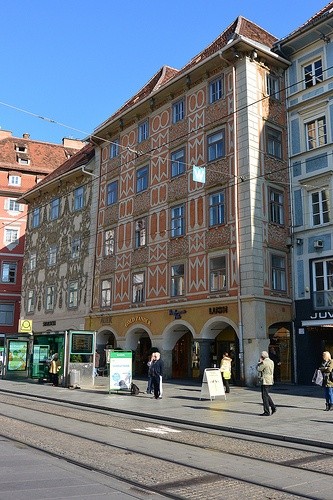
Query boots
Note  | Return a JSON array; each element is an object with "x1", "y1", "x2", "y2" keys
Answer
[{"x1": 324, "y1": 402, "x2": 333, "y2": 411}]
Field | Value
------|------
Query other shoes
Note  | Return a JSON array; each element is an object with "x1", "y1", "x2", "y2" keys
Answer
[
  {"x1": 260, "y1": 413, "x2": 270, "y2": 416},
  {"x1": 271, "y1": 408, "x2": 277, "y2": 414},
  {"x1": 151, "y1": 397, "x2": 155, "y2": 399},
  {"x1": 157, "y1": 397, "x2": 162, "y2": 399},
  {"x1": 225, "y1": 391, "x2": 229, "y2": 393},
  {"x1": 143, "y1": 391, "x2": 147, "y2": 394}
]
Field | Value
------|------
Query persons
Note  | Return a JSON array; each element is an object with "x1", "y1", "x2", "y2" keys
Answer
[
  {"x1": 151, "y1": 352, "x2": 163, "y2": 399},
  {"x1": 319, "y1": 351, "x2": 333, "y2": 411},
  {"x1": 47, "y1": 350, "x2": 61, "y2": 386},
  {"x1": 146, "y1": 356, "x2": 154, "y2": 394},
  {"x1": 94, "y1": 349, "x2": 99, "y2": 376},
  {"x1": 219, "y1": 352, "x2": 232, "y2": 394},
  {"x1": 256, "y1": 350, "x2": 277, "y2": 416}
]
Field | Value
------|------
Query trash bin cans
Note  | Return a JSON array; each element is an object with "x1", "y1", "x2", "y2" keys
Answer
[{"x1": 69, "y1": 369, "x2": 80, "y2": 386}]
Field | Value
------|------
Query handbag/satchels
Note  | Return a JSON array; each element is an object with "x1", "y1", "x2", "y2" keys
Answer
[{"x1": 312, "y1": 370, "x2": 324, "y2": 386}]
